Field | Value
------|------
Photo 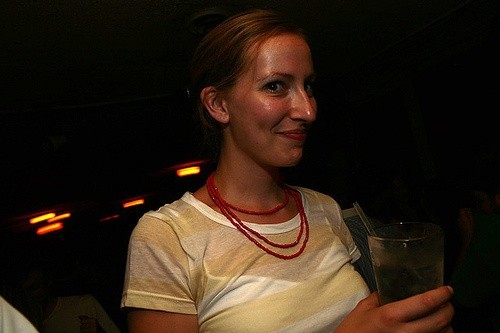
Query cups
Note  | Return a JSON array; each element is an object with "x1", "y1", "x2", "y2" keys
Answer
[{"x1": 368, "y1": 221, "x2": 443, "y2": 307}]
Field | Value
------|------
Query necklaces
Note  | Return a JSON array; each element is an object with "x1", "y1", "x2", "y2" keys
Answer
[{"x1": 205, "y1": 175, "x2": 310, "y2": 259}]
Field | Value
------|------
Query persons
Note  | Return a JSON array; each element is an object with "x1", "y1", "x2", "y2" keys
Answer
[
  {"x1": 118, "y1": 9, "x2": 454, "y2": 333},
  {"x1": 0, "y1": 254, "x2": 120, "y2": 333}
]
